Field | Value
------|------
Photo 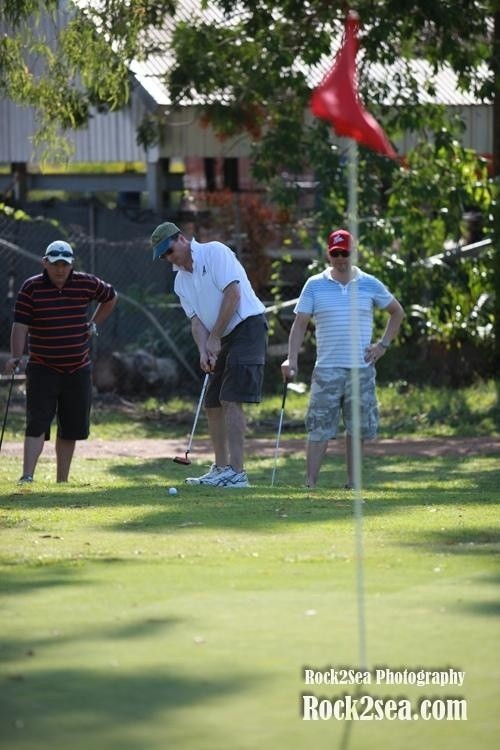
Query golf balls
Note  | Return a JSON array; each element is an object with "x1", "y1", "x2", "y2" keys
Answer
[{"x1": 169, "y1": 487, "x2": 177, "y2": 495}]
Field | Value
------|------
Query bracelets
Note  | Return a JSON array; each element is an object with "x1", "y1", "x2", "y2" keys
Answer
[
  {"x1": 380, "y1": 338, "x2": 392, "y2": 351},
  {"x1": 86, "y1": 322, "x2": 98, "y2": 335}
]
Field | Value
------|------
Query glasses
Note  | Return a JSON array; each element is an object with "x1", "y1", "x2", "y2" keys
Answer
[
  {"x1": 159, "y1": 248, "x2": 175, "y2": 260},
  {"x1": 43, "y1": 249, "x2": 75, "y2": 259},
  {"x1": 329, "y1": 250, "x2": 351, "y2": 257}
]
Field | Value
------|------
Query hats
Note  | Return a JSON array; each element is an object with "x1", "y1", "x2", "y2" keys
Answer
[
  {"x1": 326, "y1": 229, "x2": 355, "y2": 252},
  {"x1": 43, "y1": 239, "x2": 75, "y2": 264},
  {"x1": 147, "y1": 221, "x2": 182, "y2": 262}
]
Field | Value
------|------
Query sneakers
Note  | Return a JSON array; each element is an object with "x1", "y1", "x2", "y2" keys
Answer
[
  {"x1": 18, "y1": 475, "x2": 34, "y2": 484},
  {"x1": 184, "y1": 463, "x2": 224, "y2": 486},
  {"x1": 202, "y1": 465, "x2": 251, "y2": 489}
]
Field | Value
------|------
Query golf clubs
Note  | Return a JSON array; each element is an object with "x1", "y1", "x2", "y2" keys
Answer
[{"x1": 174, "y1": 357, "x2": 212, "y2": 465}]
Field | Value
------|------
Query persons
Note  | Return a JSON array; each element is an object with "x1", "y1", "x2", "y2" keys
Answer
[
  {"x1": 148, "y1": 220, "x2": 270, "y2": 488},
  {"x1": 5, "y1": 239, "x2": 119, "y2": 484},
  {"x1": 280, "y1": 229, "x2": 406, "y2": 491}
]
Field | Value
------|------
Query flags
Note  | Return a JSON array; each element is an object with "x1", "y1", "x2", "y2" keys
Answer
[{"x1": 309, "y1": 7, "x2": 412, "y2": 170}]
